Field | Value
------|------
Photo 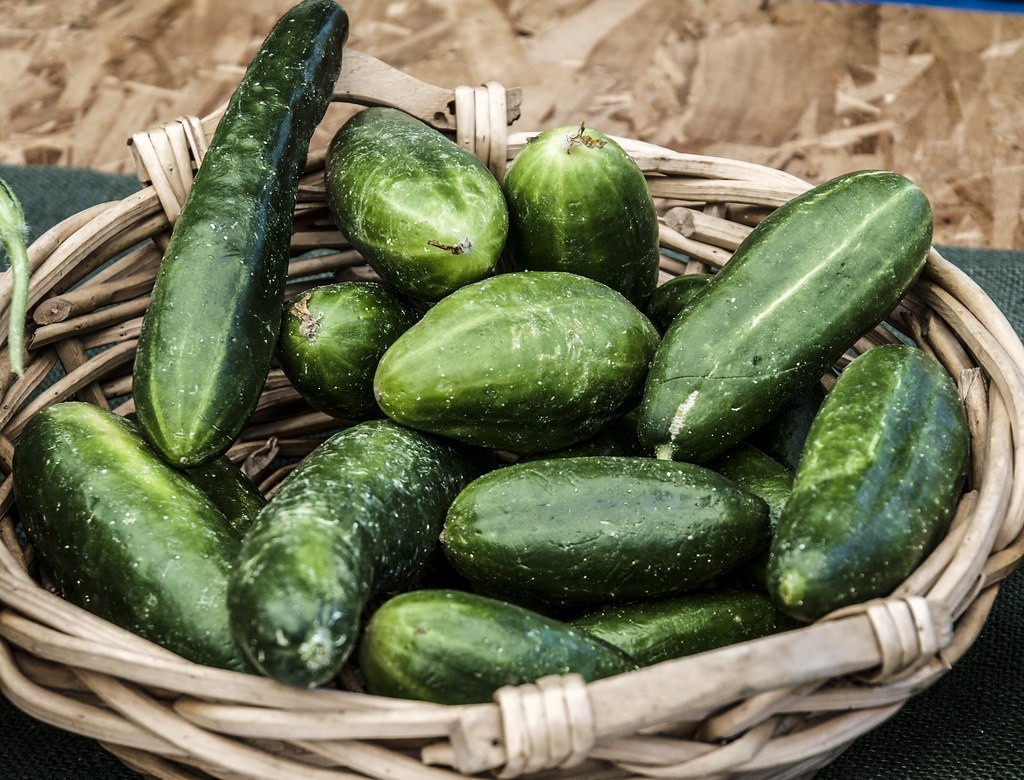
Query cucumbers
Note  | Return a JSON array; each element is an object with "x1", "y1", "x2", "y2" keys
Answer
[{"x1": 7, "y1": 0, "x2": 971, "y2": 706}]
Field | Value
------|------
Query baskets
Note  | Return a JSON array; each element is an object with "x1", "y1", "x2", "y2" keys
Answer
[{"x1": 0, "y1": 80, "x2": 1024, "y2": 780}]
